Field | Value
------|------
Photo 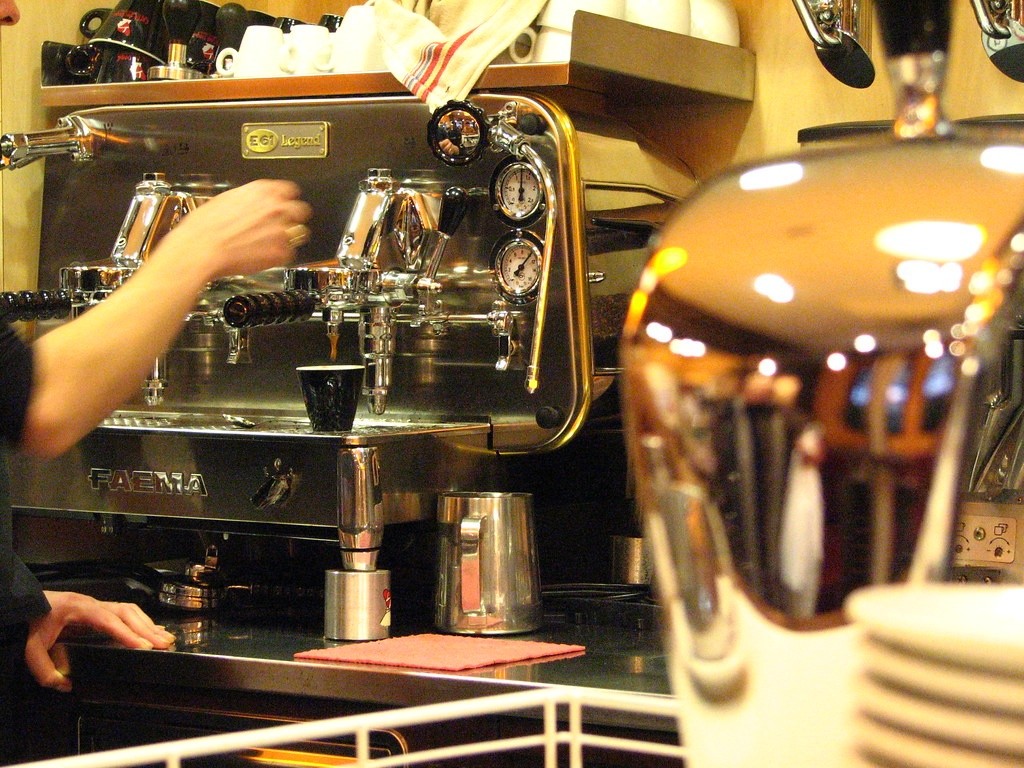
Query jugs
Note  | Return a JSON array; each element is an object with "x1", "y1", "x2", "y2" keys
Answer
[{"x1": 433, "y1": 490, "x2": 543, "y2": 636}]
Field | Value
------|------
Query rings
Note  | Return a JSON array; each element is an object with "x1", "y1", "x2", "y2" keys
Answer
[{"x1": 289, "y1": 225, "x2": 309, "y2": 248}]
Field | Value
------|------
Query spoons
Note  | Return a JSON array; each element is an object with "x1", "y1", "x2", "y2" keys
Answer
[{"x1": 222, "y1": 412, "x2": 299, "y2": 428}]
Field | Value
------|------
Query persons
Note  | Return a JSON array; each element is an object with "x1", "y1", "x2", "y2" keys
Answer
[{"x1": 0, "y1": 0, "x2": 313, "y2": 694}]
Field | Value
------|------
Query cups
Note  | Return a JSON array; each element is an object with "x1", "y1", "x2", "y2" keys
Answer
[
  {"x1": 612, "y1": 535, "x2": 649, "y2": 585},
  {"x1": 296, "y1": 365, "x2": 366, "y2": 432},
  {"x1": 37, "y1": 1, "x2": 741, "y2": 89}
]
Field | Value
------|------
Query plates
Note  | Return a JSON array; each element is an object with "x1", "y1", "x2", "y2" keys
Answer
[{"x1": 842, "y1": 583, "x2": 1023, "y2": 767}]
